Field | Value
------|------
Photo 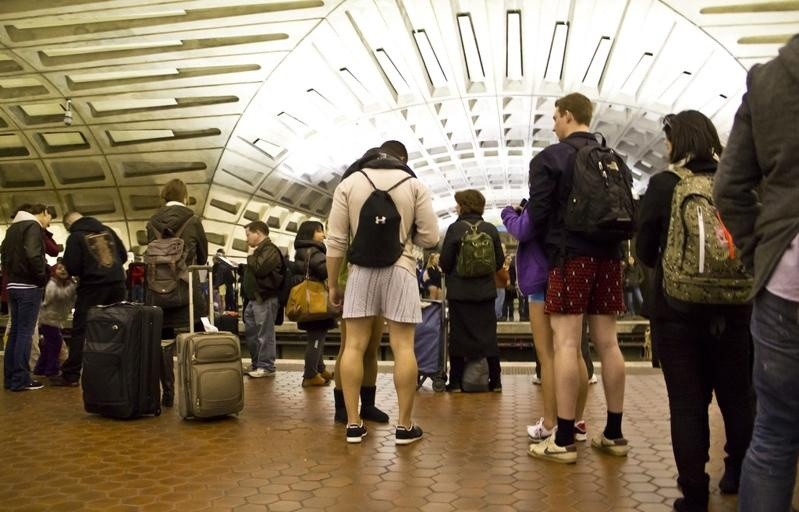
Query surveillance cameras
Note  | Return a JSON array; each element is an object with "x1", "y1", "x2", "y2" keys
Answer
[{"x1": 62, "y1": 110, "x2": 72, "y2": 127}]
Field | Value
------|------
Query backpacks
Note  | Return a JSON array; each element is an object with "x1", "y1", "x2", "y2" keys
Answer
[
  {"x1": 348, "y1": 186, "x2": 405, "y2": 267},
  {"x1": 663, "y1": 171, "x2": 754, "y2": 305},
  {"x1": 81, "y1": 230, "x2": 119, "y2": 267},
  {"x1": 456, "y1": 223, "x2": 497, "y2": 278},
  {"x1": 565, "y1": 142, "x2": 638, "y2": 240},
  {"x1": 146, "y1": 220, "x2": 189, "y2": 308}
]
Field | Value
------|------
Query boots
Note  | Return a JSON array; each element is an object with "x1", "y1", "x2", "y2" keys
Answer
[
  {"x1": 162, "y1": 345, "x2": 174, "y2": 406},
  {"x1": 360, "y1": 386, "x2": 388, "y2": 422},
  {"x1": 675, "y1": 472, "x2": 709, "y2": 512},
  {"x1": 334, "y1": 388, "x2": 347, "y2": 421},
  {"x1": 719, "y1": 457, "x2": 742, "y2": 494}
]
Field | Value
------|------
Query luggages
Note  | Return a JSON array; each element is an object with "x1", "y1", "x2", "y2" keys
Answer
[
  {"x1": 176, "y1": 265, "x2": 244, "y2": 420},
  {"x1": 82, "y1": 262, "x2": 163, "y2": 418},
  {"x1": 414, "y1": 273, "x2": 448, "y2": 391}
]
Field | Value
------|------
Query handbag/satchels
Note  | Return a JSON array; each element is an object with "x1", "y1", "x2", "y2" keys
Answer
[
  {"x1": 462, "y1": 358, "x2": 490, "y2": 393},
  {"x1": 286, "y1": 281, "x2": 332, "y2": 321},
  {"x1": 276, "y1": 306, "x2": 286, "y2": 323}
]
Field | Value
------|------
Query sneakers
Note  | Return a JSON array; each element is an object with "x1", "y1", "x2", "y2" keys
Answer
[
  {"x1": 243, "y1": 363, "x2": 275, "y2": 377},
  {"x1": 446, "y1": 383, "x2": 461, "y2": 393},
  {"x1": 395, "y1": 421, "x2": 422, "y2": 445},
  {"x1": 491, "y1": 383, "x2": 502, "y2": 392},
  {"x1": 49, "y1": 372, "x2": 79, "y2": 386},
  {"x1": 5, "y1": 377, "x2": 43, "y2": 390},
  {"x1": 303, "y1": 369, "x2": 334, "y2": 386},
  {"x1": 526, "y1": 418, "x2": 630, "y2": 464},
  {"x1": 346, "y1": 420, "x2": 367, "y2": 443}
]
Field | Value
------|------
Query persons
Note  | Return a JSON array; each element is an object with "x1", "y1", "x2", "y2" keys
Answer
[
  {"x1": 527, "y1": 93, "x2": 633, "y2": 464},
  {"x1": 236, "y1": 221, "x2": 284, "y2": 378},
  {"x1": 502, "y1": 200, "x2": 598, "y2": 440},
  {"x1": 441, "y1": 189, "x2": 505, "y2": 393},
  {"x1": 420, "y1": 253, "x2": 443, "y2": 300},
  {"x1": 623, "y1": 256, "x2": 645, "y2": 315},
  {"x1": 712, "y1": 35, "x2": 799, "y2": 512},
  {"x1": 294, "y1": 221, "x2": 334, "y2": 387},
  {"x1": 325, "y1": 140, "x2": 440, "y2": 445},
  {"x1": 212, "y1": 249, "x2": 235, "y2": 311},
  {"x1": 33, "y1": 261, "x2": 77, "y2": 377},
  {"x1": 494, "y1": 241, "x2": 529, "y2": 319},
  {"x1": 49, "y1": 212, "x2": 127, "y2": 386},
  {"x1": 635, "y1": 109, "x2": 757, "y2": 512},
  {"x1": 143, "y1": 179, "x2": 209, "y2": 406},
  {"x1": 0, "y1": 203, "x2": 60, "y2": 391}
]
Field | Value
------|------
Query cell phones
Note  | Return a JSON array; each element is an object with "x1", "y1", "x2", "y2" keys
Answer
[{"x1": 515, "y1": 198, "x2": 528, "y2": 216}]
